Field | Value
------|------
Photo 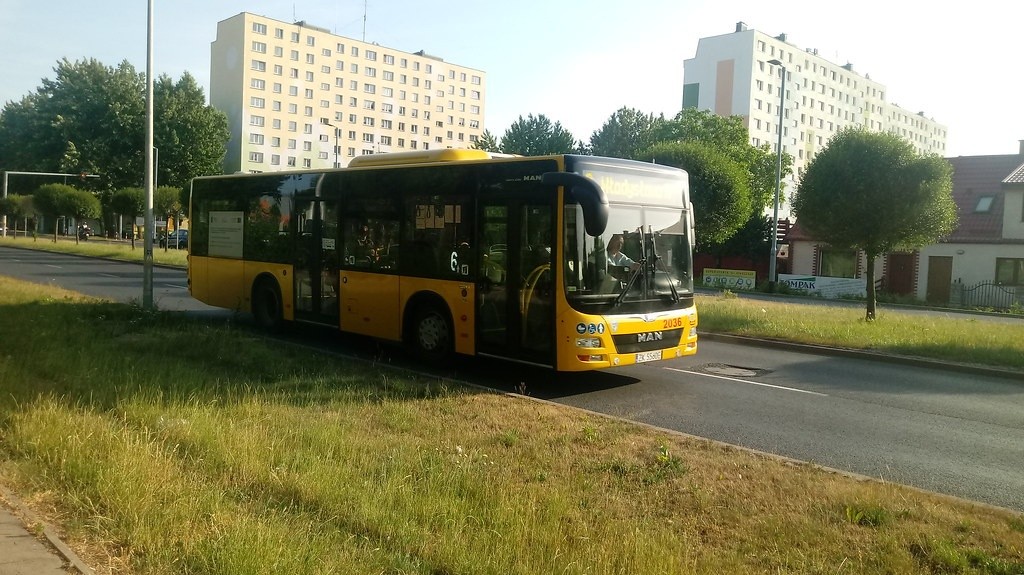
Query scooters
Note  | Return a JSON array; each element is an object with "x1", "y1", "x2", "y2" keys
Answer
[{"x1": 79, "y1": 227, "x2": 91, "y2": 241}]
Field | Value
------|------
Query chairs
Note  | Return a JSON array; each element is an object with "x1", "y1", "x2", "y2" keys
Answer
[{"x1": 488, "y1": 244, "x2": 507, "y2": 260}]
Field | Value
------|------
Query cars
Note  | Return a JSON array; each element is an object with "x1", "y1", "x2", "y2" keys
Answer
[{"x1": 159, "y1": 229, "x2": 189, "y2": 249}]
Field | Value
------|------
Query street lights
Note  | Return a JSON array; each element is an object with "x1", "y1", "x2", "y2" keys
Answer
[
  {"x1": 326, "y1": 123, "x2": 339, "y2": 168},
  {"x1": 152, "y1": 146, "x2": 159, "y2": 240},
  {"x1": 766, "y1": 59, "x2": 785, "y2": 294}
]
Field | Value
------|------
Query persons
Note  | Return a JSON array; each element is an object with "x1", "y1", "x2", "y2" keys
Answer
[
  {"x1": 356, "y1": 225, "x2": 379, "y2": 264},
  {"x1": 82, "y1": 222, "x2": 88, "y2": 232},
  {"x1": 454, "y1": 207, "x2": 486, "y2": 281},
  {"x1": 596, "y1": 235, "x2": 642, "y2": 276}
]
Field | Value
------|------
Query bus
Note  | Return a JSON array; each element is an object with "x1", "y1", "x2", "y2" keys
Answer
[{"x1": 184, "y1": 148, "x2": 698, "y2": 374}]
